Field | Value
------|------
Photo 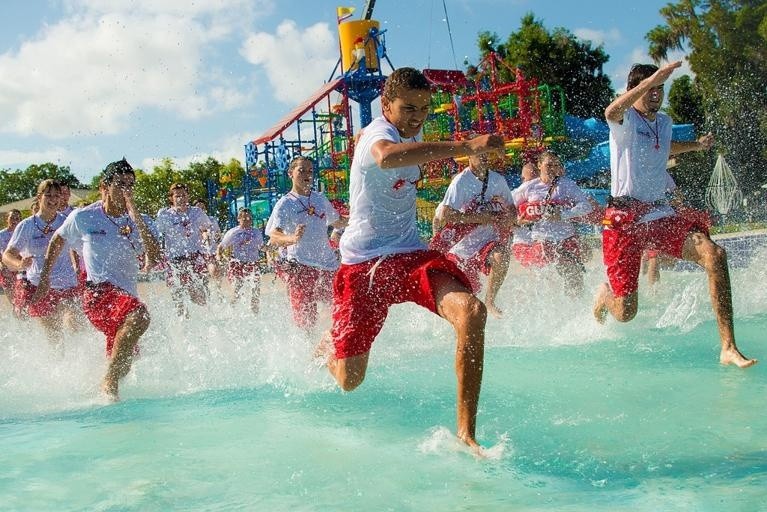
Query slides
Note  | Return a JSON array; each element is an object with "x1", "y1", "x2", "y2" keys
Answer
[{"x1": 229, "y1": 192, "x2": 286, "y2": 224}]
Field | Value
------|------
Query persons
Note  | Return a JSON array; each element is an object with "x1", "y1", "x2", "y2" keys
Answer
[
  {"x1": 32, "y1": 160, "x2": 158, "y2": 402},
  {"x1": 594, "y1": 64, "x2": 757, "y2": 367},
  {"x1": 265, "y1": 155, "x2": 343, "y2": 330},
  {"x1": 430, "y1": 132, "x2": 594, "y2": 319},
  {"x1": 313, "y1": 66, "x2": 489, "y2": 454},
  {"x1": 1, "y1": 177, "x2": 287, "y2": 334}
]
